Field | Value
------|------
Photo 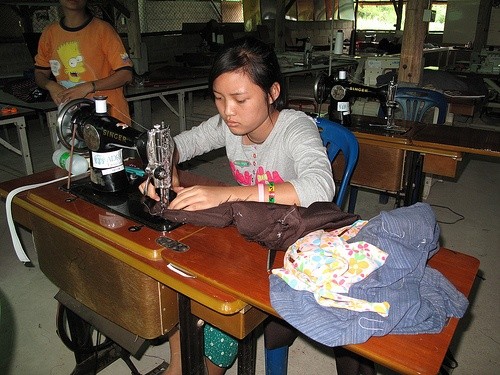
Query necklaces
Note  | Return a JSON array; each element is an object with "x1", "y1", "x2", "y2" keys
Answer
[{"x1": 246, "y1": 134, "x2": 266, "y2": 145}]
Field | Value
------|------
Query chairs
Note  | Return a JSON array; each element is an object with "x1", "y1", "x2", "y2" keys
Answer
[
  {"x1": 312, "y1": 119, "x2": 359, "y2": 211},
  {"x1": 376, "y1": 87, "x2": 449, "y2": 205}
]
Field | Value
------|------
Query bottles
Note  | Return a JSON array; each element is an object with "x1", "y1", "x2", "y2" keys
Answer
[{"x1": 52, "y1": 149, "x2": 89, "y2": 174}]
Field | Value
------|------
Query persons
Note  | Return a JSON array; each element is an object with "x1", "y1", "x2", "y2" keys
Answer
[
  {"x1": 33, "y1": 0, "x2": 135, "y2": 127},
  {"x1": 138, "y1": 37, "x2": 335, "y2": 375}
]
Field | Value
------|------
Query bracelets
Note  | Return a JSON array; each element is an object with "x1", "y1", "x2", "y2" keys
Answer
[
  {"x1": 91, "y1": 81, "x2": 96, "y2": 94},
  {"x1": 259, "y1": 184, "x2": 265, "y2": 202},
  {"x1": 269, "y1": 182, "x2": 276, "y2": 203}
]
